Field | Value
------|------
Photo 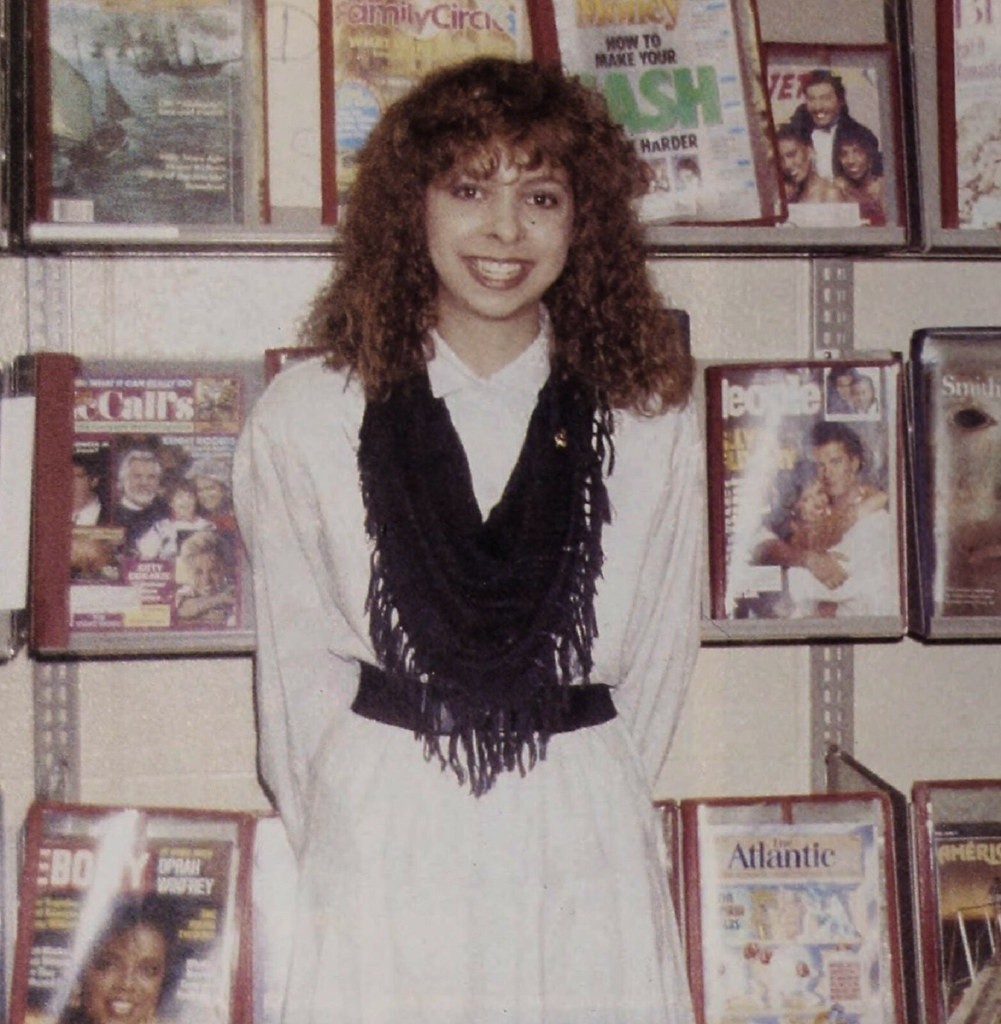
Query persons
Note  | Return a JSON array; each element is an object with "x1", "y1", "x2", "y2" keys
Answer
[
  {"x1": 228, "y1": 54, "x2": 712, "y2": 1024},
  {"x1": 75, "y1": 429, "x2": 233, "y2": 633},
  {"x1": 71, "y1": 909, "x2": 162, "y2": 1024},
  {"x1": 768, "y1": 66, "x2": 882, "y2": 228},
  {"x1": 732, "y1": 372, "x2": 892, "y2": 621}
]
